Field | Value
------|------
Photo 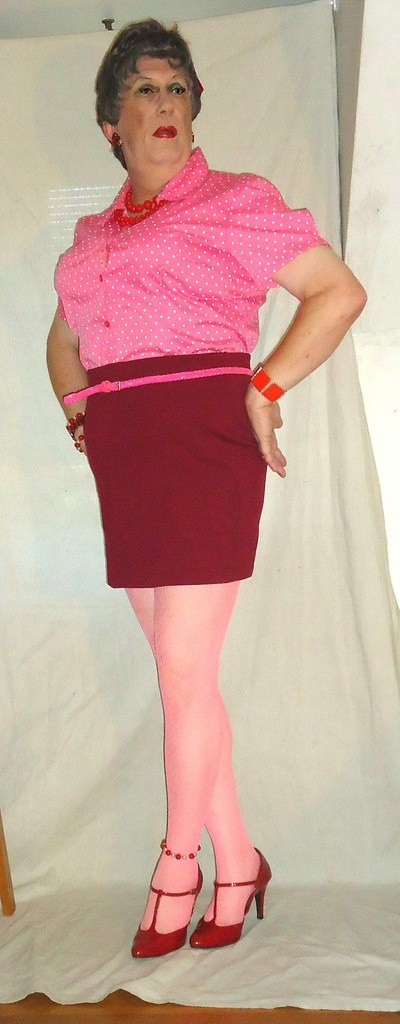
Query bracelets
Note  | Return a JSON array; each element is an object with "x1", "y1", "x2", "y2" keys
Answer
[
  {"x1": 66, "y1": 411, "x2": 85, "y2": 452},
  {"x1": 250, "y1": 361, "x2": 286, "y2": 402}
]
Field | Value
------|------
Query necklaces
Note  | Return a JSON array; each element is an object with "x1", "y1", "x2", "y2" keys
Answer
[{"x1": 114, "y1": 191, "x2": 168, "y2": 228}]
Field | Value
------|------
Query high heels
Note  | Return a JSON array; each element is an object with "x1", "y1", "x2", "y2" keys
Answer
[
  {"x1": 131, "y1": 859, "x2": 203, "y2": 959},
  {"x1": 189, "y1": 846, "x2": 272, "y2": 948}
]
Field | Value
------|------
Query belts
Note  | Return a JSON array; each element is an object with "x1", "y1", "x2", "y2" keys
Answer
[{"x1": 63, "y1": 368, "x2": 253, "y2": 405}]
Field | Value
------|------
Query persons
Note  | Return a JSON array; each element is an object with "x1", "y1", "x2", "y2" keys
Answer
[{"x1": 44, "y1": 17, "x2": 369, "y2": 961}]
